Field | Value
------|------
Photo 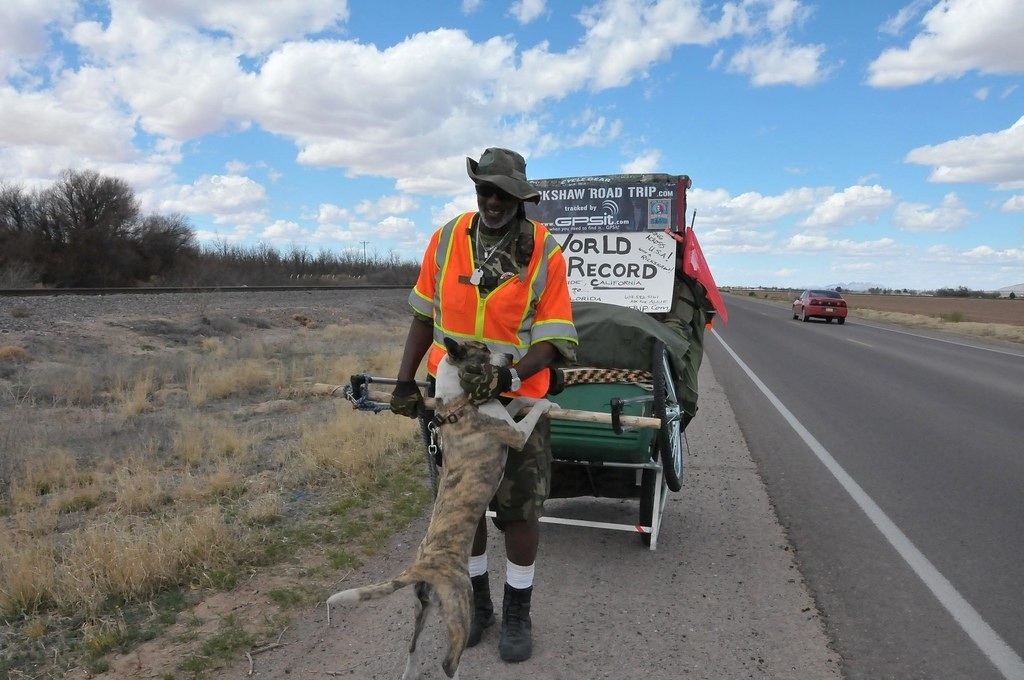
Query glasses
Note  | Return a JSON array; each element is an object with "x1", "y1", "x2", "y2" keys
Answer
[{"x1": 475, "y1": 184, "x2": 515, "y2": 203}]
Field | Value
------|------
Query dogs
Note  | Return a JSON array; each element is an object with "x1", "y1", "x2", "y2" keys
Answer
[{"x1": 316, "y1": 338, "x2": 561, "y2": 680}]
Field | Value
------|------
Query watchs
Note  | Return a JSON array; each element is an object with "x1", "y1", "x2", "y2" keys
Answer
[{"x1": 509, "y1": 367, "x2": 521, "y2": 393}]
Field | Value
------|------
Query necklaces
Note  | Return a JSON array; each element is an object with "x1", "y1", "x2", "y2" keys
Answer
[{"x1": 470, "y1": 218, "x2": 512, "y2": 285}]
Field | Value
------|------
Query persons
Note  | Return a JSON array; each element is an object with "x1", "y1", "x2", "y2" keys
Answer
[{"x1": 389, "y1": 149, "x2": 578, "y2": 662}]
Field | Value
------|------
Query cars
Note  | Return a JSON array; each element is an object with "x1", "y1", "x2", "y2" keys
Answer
[{"x1": 792, "y1": 289, "x2": 849, "y2": 324}]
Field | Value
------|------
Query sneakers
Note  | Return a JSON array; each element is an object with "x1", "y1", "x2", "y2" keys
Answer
[
  {"x1": 499, "y1": 582, "x2": 534, "y2": 662},
  {"x1": 465, "y1": 571, "x2": 495, "y2": 648}
]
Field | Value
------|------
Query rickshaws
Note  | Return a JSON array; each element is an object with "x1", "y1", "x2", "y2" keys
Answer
[{"x1": 314, "y1": 172, "x2": 728, "y2": 550}]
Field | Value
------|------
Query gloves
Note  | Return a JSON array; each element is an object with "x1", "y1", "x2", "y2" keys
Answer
[
  {"x1": 458, "y1": 363, "x2": 513, "y2": 406},
  {"x1": 390, "y1": 380, "x2": 424, "y2": 419}
]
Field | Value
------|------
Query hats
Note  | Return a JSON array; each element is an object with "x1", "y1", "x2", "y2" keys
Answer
[{"x1": 467, "y1": 148, "x2": 541, "y2": 206}]
